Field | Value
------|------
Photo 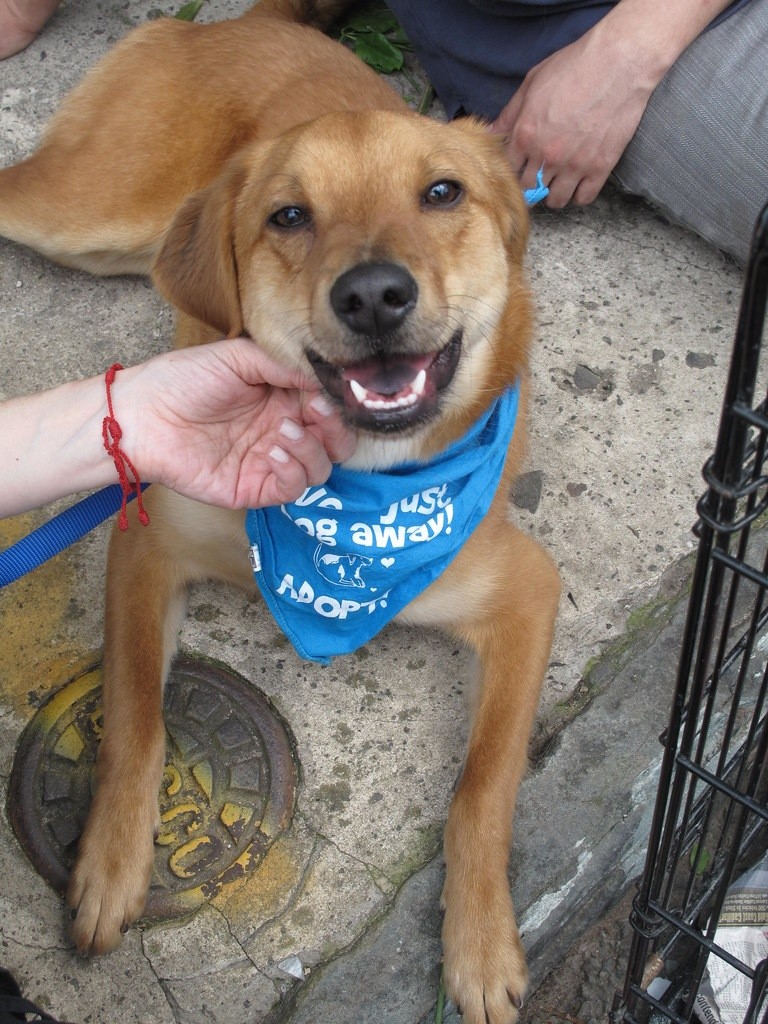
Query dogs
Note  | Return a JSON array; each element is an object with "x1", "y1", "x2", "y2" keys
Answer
[{"x1": 0, "y1": 16, "x2": 560, "y2": 1024}]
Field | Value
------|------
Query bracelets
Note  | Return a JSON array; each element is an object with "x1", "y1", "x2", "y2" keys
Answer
[{"x1": 103, "y1": 364, "x2": 152, "y2": 529}]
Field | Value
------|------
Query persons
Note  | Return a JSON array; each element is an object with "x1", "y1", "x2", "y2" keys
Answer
[
  {"x1": 0, "y1": 0, "x2": 768, "y2": 270},
  {"x1": 0, "y1": 339, "x2": 357, "y2": 510}
]
